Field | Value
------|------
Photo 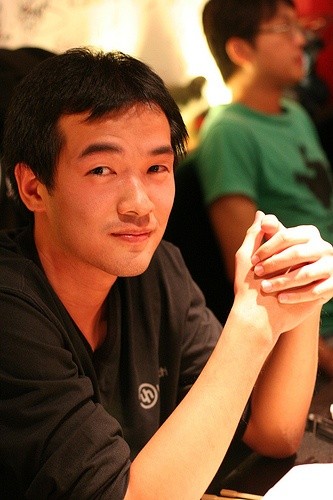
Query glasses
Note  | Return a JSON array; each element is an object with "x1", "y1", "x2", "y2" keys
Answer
[{"x1": 255, "y1": 22, "x2": 293, "y2": 37}]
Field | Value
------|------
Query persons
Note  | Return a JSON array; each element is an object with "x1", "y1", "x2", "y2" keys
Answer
[
  {"x1": 190, "y1": 0, "x2": 333, "y2": 395},
  {"x1": 1, "y1": 45, "x2": 333, "y2": 500}
]
